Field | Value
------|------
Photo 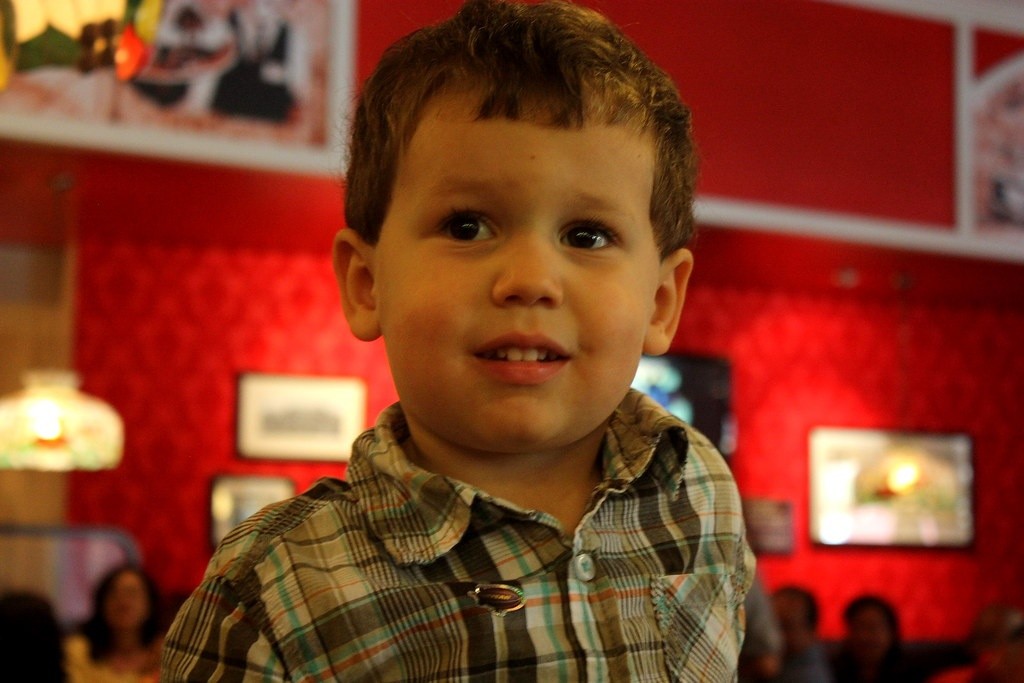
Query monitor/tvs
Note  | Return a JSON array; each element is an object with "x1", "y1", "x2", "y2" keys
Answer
[{"x1": 630, "y1": 352, "x2": 732, "y2": 470}]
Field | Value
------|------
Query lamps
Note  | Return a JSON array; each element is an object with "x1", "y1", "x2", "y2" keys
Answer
[{"x1": 0, "y1": 173, "x2": 126, "y2": 472}]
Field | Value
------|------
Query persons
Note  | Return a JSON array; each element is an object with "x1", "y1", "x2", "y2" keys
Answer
[
  {"x1": 743, "y1": 583, "x2": 1024, "y2": 683},
  {"x1": 62, "y1": 567, "x2": 166, "y2": 683},
  {"x1": 164, "y1": 1, "x2": 757, "y2": 683}
]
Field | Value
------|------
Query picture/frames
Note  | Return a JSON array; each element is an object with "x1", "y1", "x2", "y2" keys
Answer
[{"x1": 0, "y1": 0, "x2": 358, "y2": 177}]
